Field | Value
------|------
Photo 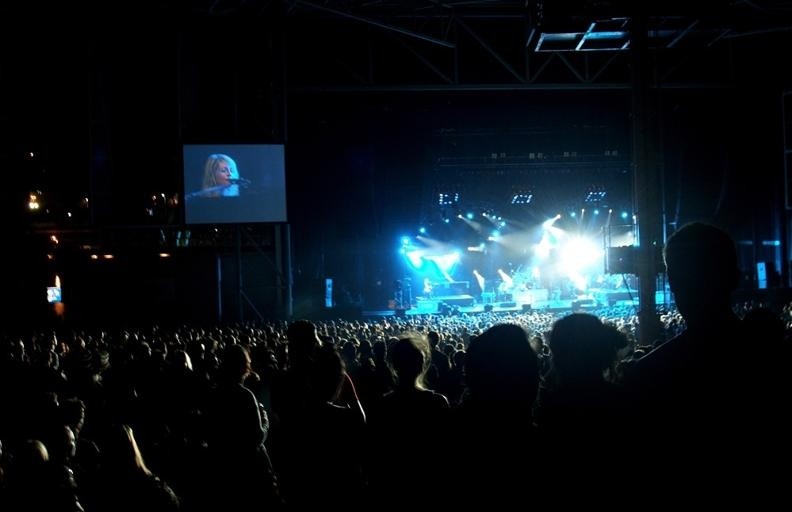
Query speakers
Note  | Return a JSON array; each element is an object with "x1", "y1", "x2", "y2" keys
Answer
[
  {"x1": 757, "y1": 261, "x2": 774, "y2": 289},
  {"x1": 523, "y1": 304, "x2": 531, "y2": 309},
  {"x1": 608, "y1": 246, "x2": 665, "y2": 274}
]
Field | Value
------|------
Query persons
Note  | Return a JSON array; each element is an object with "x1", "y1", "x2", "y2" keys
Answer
[
  {"x1": 1, "y1": 218, "x2": 790, "y2": 511},
  {"x1": 196, "y1": 155, "x2": 239, "y2": 198}
]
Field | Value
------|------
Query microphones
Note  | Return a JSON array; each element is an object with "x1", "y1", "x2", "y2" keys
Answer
[{"x1": 230, "y1": 178, "x2": 251, "y2": 185}]
